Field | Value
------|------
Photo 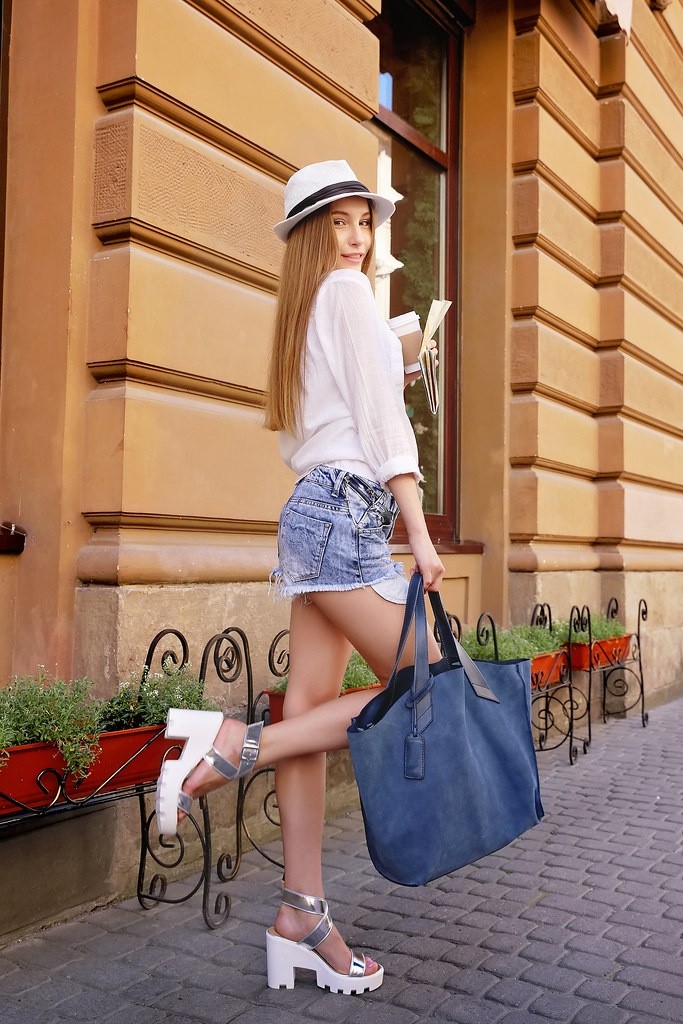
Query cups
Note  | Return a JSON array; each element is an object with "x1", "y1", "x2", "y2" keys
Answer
[{"x1": 386, "y1": 310, "x2": 423, "y2": 374}]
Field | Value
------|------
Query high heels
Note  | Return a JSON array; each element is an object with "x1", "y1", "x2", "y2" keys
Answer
[
  {"x1": 157, "y1": 706, "x2": 262, "y2": 836},
  {"x1": 266, "y1": 889, "x2": 383, "y2": 995}
]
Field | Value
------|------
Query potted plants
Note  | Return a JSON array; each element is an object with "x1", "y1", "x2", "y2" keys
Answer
[
  {"x1": 453, "y1": 613, "x2": 632, "y2": 692},
  {"x1": 262, "y1": 650, "x2": 388, "y2": 729},
  {"x1": 0, "y1": 652, "x2": 225, "y2": 815}
]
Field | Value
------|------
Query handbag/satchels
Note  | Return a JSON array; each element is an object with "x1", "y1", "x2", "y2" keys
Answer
[{"x1": 348, "y1": 571, "x2": 547, "y2": 885}]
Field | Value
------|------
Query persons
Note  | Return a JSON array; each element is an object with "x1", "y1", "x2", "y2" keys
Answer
[{"x1": 158, "y1": 159, "x2": 446, "y2": 990}]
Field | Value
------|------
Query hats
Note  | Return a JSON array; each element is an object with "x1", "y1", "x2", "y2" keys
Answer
[{"x1": 274, "y1": 160, "x2": 395, "y2": 245}]
